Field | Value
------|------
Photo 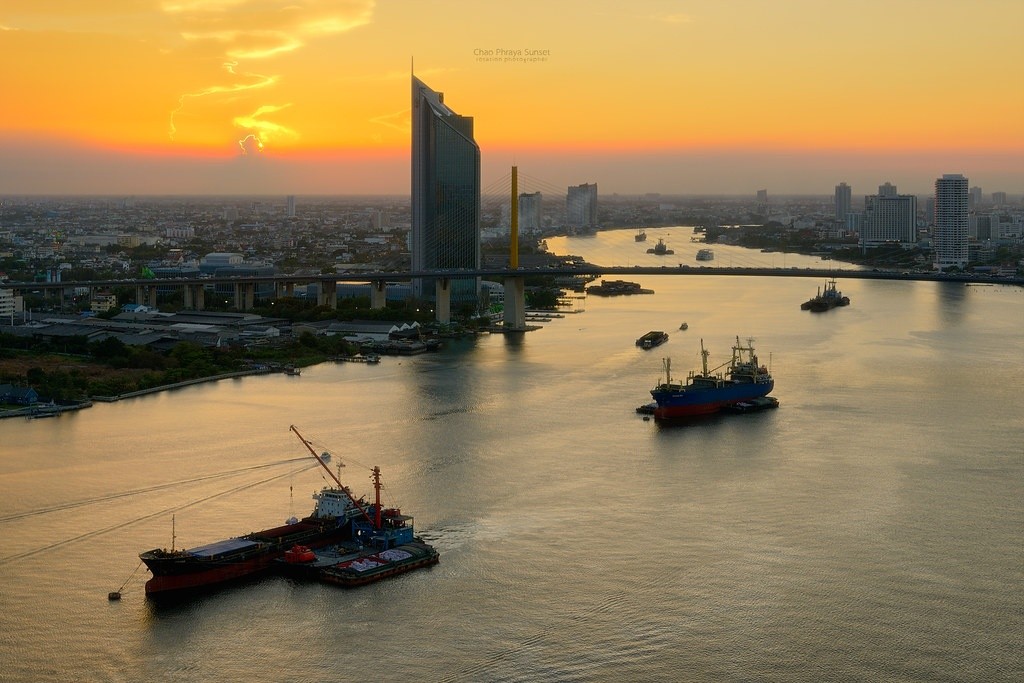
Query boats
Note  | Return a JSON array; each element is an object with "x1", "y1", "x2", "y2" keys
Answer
[
  {"x1": 635, "y1": 335, "x2": 780, "y2": 425},
  {"x1": 634, "y1": 229, "x2": 647, "y2": 242},
  {"x1": 635, "y1": 331, "x2": 669, "y2": 349},
  {"x1": 646, "y1": 236, "x2": 675, "y2": 254},
  {"x1": 696, "y1": 248, "x2": 715, "y2": 261},
  {"x1": 137, "y1": 423, "x2": 441, "y2": 609},
  {"x1": 800, "y1": 276, "x2": 850, "y2": 313}
]
